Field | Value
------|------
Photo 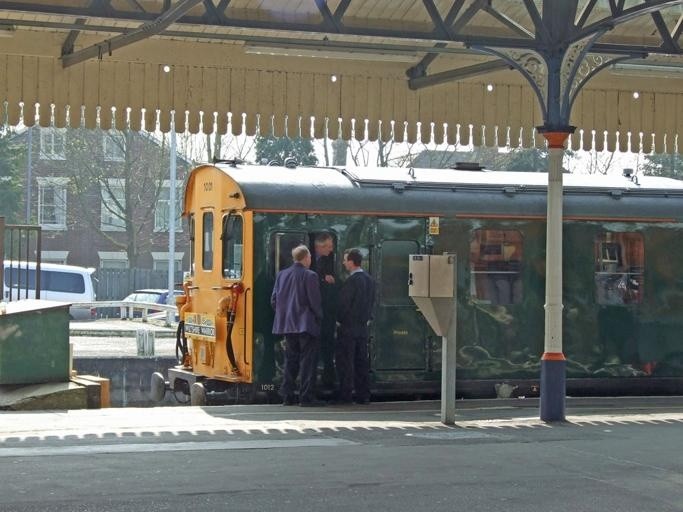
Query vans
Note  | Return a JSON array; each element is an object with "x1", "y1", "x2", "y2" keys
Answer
[{"x1": 11, "y1": 261, "x2": 96, "y2": 319}]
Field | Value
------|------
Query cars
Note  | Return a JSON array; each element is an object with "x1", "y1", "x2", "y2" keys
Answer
[{"x1": 121, "y1": 288, "x2": 184, "y2": 319}]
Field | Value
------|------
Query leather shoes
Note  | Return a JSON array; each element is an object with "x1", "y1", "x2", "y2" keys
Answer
[{"x1": 284, "y1": 383, "x2": 370, "y2": 407}]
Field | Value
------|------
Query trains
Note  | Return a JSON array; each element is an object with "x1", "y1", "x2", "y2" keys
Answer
[{"x1": 151, "y1": 156, "x2": 682, "y2": 406}]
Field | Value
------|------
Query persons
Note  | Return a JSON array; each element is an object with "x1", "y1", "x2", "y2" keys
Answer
[
  {"x1": 310, "y1": 233, "x2": 336, "y2": 383},
  {"x1": 333, "y1": 248, "x2": 376, "y2": 405},
  {"x1": 269, "y1": 243, "x2": 324, "y2": 406}
]
